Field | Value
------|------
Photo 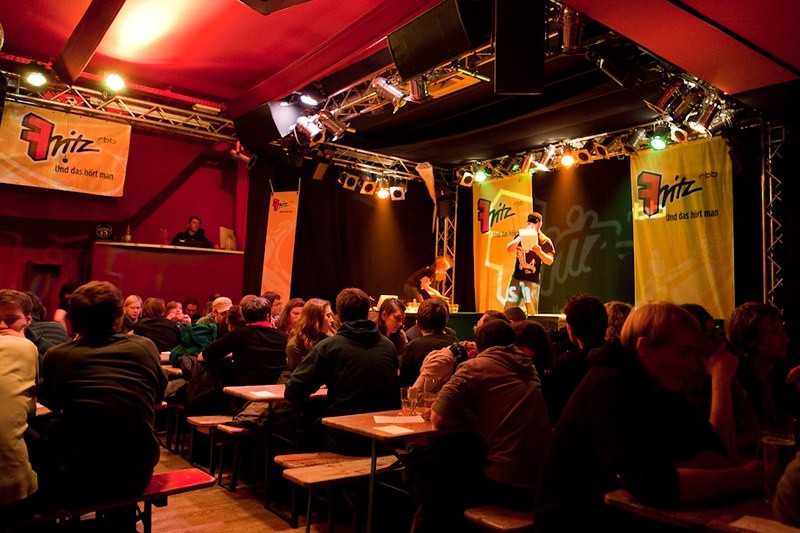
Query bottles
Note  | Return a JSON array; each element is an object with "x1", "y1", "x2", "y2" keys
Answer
[{"x1": 124, "y1": 224, "x2": 132, "y2": 243}]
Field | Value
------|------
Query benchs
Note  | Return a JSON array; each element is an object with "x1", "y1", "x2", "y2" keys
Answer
[
  {"x1": 0, "y1": 469, "x2": 216, "y2": 533},
  {"x1": 218, "y1": 425, "x2": 253, "y2": 490},
  {"x1": 283, "y1": 454, "x2": 401, "y2": 533},
  {"x1": 273, "y1": 452, "x2": 370, "y2": 529},
  {"x1": 186, "y1": 415, "x2": 234, "y2": 475},
  {"x1": 464, "y1": 505, "x2": 551, "y2": 533}
]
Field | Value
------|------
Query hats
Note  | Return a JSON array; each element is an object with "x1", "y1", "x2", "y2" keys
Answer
[
  {"x1": 212, "y1": 296, "x2": 234, "y2": 314},
  {"x1": 526, "y1": 212, "x2": 542, "y2": 224}
]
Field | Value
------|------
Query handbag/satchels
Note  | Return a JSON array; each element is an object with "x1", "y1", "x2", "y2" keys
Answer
[{"x1": 233, "y1": 402, "x2": 271, "y2": 427}]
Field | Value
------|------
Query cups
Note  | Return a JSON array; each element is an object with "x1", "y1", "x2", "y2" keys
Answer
[
  {"x1": 422, "y1": 392, "x2": 437, "y2": 420},
  {"x1": 400, "y1": 387, "x2": 418, "y2": 416},
  {"x1": 761, "y1": 431, "x2": 793, "y2": 503}
]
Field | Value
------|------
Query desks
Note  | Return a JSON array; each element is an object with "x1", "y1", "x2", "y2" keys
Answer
[{"x1": 161, "y1": 365, "x2": 800, "y2": 533}]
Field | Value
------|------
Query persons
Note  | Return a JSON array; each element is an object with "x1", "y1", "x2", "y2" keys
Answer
[
  {"x1": 530, "y1": 299, "x2": 763, "y2": 533},
  {"x1": 166, "y1": 287, "x2": 408, "y2": 507},
  {"x1": 406, "y1": 294, "x2": 631, "y2": 533},
  {"x1": 121, "y1": 295, "x2": 143, "y2": 335},
  {"x1": 0, "y1": 289, "x2": 55, "y2": 372},
  {"x1": 23, "y1": 285, "x2": 81, "y2": 346},
  {"x1": 504, "y1": 212, "x2": 555, "y2": 315},
  {"x1": 0, "y1": 336, "x2": 38, "y2": 528},
  {"x1": 404, "y1": 258, "x2": 450, "y2": 303},
  {"x1": 40, "y1": 280, "x2": 168, "y2": 533},
  {"x1": 171, "y1": 216, "x2": 211, "y2": 245},
  {"x1": 133, "y1": 298, "x2": 182, "y2": 354},
  {"x1": 679, "y1": 302, "x2": 800, "y2": 520}
]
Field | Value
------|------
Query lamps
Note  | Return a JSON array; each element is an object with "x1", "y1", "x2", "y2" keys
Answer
[
  {"x1": 230, "y1": 141, "x2": 257, "y2": 169},
  {"x1": 288, "y1": 145, "x2": 407, "y2": 200},
  {"x1": 644, "y1": 69, "x2": 762, "y2": 151},
  {"x1": 457, "y1": 133, "x2": 621, "y2": 187},
  {"x1": 289, "y1": 90, "x2": 356, "y2": 148},
  {"x1": 372, "y1": 74, "x2": 433, "y2": 114},
  {"x1": 545, "y1": 0, "x2": 589, "y2": 56},
  {"x1": 625, "y1": 128, "x2": 646, "y2": 153}
]
